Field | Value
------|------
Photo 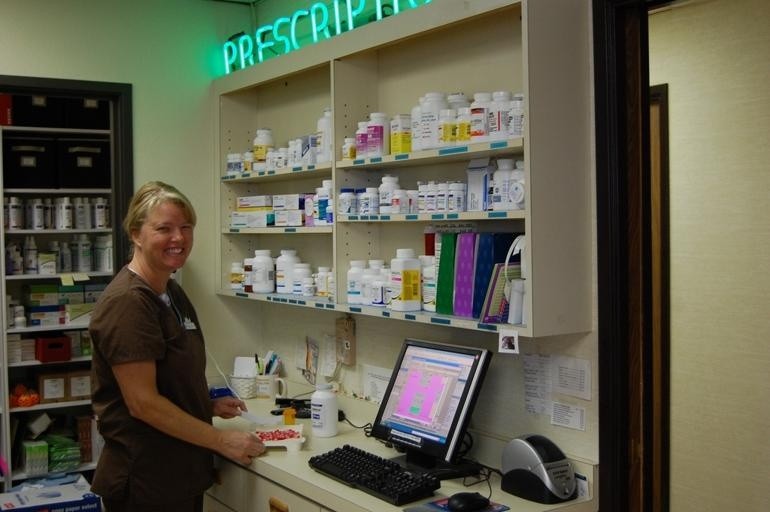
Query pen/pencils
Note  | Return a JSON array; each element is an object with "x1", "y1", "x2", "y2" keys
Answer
[
  {"x1": 270, "y1": 359, "x2": 280, "y2": 377},
  {"x1": 266, "y1": 360, "x2": 273, "y2": 376},
  {"x1": 272, "y1": 353, "x2": 278, "y2": 364},
  {"x1": 276, "y1": 357, "x2": 283, "y2": 375},
  {"x1": 259, "y1": 359, "x2": 264, "y2": 376},
  {"x1": 255, "y1": 354, "x2": 260, "y2": 376}
]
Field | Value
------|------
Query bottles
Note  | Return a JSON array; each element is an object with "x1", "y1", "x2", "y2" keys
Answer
[
  {"x1": 7, "y1": 235, "x2": 37, "y2": 274},
  {"x1": 491, "y1": 158, "x2": 515, "y2": 210},
  {"x1": 508, "y1": 177, "x2": 525, "y2": 210},
  {"x1": 366, "y1": 112, "x2": 390, "y2": 157},
  {"x1": 345, "y1": 248, "x2": 435, "y2": 314},
  {"x1": 409, "y1": 90, "x2": 524, "y2": 151},
  {"x1": 265, "y1": 138, "x2": 302, "y2": 167},
  {"x1": 252, "y1": 128, "x2": 273, "y2": 170},
  {"x1": 341, "y1": 137, "x2": 355, "y2": 160},
  {"x1": 354, "y1": 121, "x2": 368, "y2": 158},
  {"x1": 229, "y1": 249, "x2": 332, "y2": 297},
  {"x1": 226, "y1": 152, "x2": 253, "y2": 176},
  {"x1": 48, "y1": 233, "x2": 112, "y2": 273},
  {"x1": 2, "y1": 196, "x2": 109, "y2": 229},
  {"x1": 339, "y1": 174, "x2": 467, "y2": 213},
  {"x1": 508, "y1": 160, "x2": 525, "y2": 210},
  {"x1": 317, "y1": 193, "x2": 328, "y2": 225},
  {"x1": 326, "y1": 199, "x2": 332, "y2": 224},
  {"x1": 315, "y1": 107, "x2": 330, "y2": 163},
  {"x1": 310, "y1": 383, "x2": 338, "y2": 438}
]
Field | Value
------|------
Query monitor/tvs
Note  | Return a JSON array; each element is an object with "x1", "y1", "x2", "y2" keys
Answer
[{"x1": 371, "y1": 338, "x2": 495, "y2": 481}]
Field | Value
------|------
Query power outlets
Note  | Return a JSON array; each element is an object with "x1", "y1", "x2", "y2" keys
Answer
[{"x1": 333, "y1": 318, "x2": 356, "y2": 367}]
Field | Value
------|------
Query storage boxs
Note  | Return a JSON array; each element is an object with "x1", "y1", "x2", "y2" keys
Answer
[
  {"x1": 39, "y1": 370, "x2": 94, "y2": 402},
  {"x1": 36, "y1": 337, "x2": 71, "y2": 362}
]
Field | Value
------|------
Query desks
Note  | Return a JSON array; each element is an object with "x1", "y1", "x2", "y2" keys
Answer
[{"x1": 201, "y1": 396, "x2": 598, "y2": 512}]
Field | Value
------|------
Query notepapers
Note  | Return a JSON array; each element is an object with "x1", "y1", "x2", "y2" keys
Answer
[
  {"x1": 73, "y1": 273, "x2": 89, "y2": 281},
  {"x1": 60, "y1": 274, "x2": 74, "y2": 286}
]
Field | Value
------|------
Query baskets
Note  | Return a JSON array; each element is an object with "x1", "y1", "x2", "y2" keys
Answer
[{"x1": 228, "y1": 373, "x2": 257, "y2": 399}]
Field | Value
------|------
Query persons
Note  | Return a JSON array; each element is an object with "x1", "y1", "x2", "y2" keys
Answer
[{"x1": 87, "y1": 180, "x2": 266, "y2": 512}]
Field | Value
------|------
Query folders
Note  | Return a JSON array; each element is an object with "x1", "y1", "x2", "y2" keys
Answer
[
  {"x1": 435, "y1": 232, "x2": 456, "y2": 315},
  {"x1": 453, "y1": 233, "x2": 476, "y2": 316},
  {"x1": 472, "y1": 231, "x2": 525, "y2": 318}
]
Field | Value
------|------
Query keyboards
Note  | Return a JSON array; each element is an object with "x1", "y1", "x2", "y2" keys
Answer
[{"x1": 307, "y1": 444, "x2": 442, "y2": 508}]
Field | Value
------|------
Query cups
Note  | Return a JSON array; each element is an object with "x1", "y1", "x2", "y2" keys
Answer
[{"x1": 256, "y1": 375, "x2": 287, "y2": 399}]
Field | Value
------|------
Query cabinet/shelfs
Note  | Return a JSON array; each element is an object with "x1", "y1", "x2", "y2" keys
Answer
[
  {"x1": 210, "y1": 39, "x2": 332, "y2": 308},
  {"x1": 0, "y1": 125, "x2": 110, "y2": 486},
  {"x1": 336, "y1": 1, "x2": 595, "y2": 335}
]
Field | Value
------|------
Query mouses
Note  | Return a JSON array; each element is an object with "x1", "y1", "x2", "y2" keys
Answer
[{"x1": 447, "y1": 492, "x2": 491, "y2": 512}]
